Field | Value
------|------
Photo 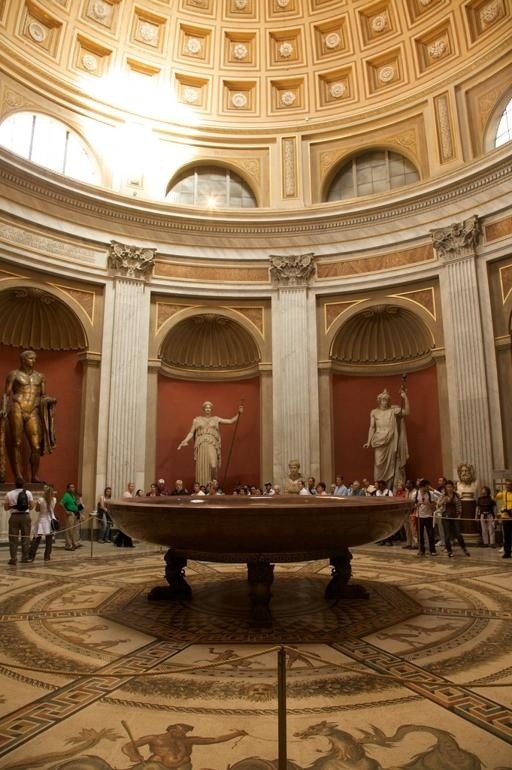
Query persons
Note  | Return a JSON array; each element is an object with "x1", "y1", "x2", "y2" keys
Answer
[
  {"x1": 0, "y1": 349, "x2": 59, "y2": 484},
  {"x1": 59, "y1": 481, "x2": 85, "y2": 552},
  {"x1": 123, "y1": 723, "x2": 248, "y2": 770},
  {"x1": 364, "y1": 476, "x2": 470, "y2": 558},
  {"x1": 476, "y1": 479, "x2": 511, "y2": 558},
  {"x1": 296, "y1": 476, "x2": 363, "y2": 496},
  {"x1": 362, "y1": 386, "x2": 412, "y2": 493},
  {"x1": 454, "y1": 461, "x2": 479, "y2": 499},
  {"x1": 137, "y1": 478, "x2": 281, "y2": 497},
  {"x1": 3, "y1": 477, "x2": 36, "y2": 566},
  {"x1": 100, "y1": 482, "x2": 135, "y2": 548},
  {"x1": 27, "y1": 484, "x2": 59, "y2": 562},
  {"x1": 284, "y1": 460, "x2": 306, "y2": 495},
  {"x1": 175, "y1": 400, "x2": 247, "y2": 486}
]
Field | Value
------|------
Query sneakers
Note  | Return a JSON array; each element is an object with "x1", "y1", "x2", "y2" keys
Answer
[{"x1": 8, "y1": 556, "x2": 51, "y2": 565}]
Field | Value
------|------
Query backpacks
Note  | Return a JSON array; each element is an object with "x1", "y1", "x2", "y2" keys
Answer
[{"x1": 17, "y1": 488, "x2": 29, "y2": 511}]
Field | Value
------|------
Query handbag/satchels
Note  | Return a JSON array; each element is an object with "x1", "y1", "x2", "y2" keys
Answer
[{"x1": 51, "y1": 519, "x2": 59, "y2": 530}]
